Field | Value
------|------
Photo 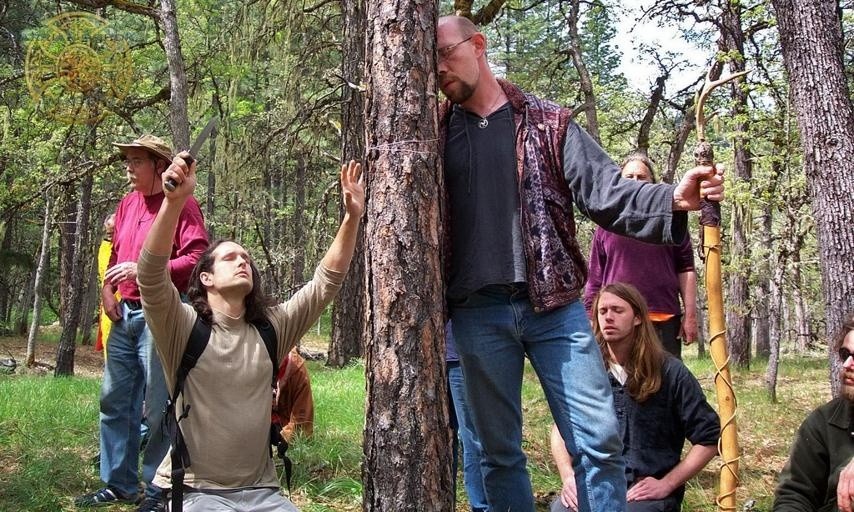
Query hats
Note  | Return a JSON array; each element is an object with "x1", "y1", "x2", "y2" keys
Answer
[{"x1": 111, "y1": 135, "x2": 172, "y2": 164}]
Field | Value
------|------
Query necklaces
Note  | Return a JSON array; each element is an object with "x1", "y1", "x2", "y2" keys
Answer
[{"x1": 478, "y1": 87, "x2": 503, "y2": 130}]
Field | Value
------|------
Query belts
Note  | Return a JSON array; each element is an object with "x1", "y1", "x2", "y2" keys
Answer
[{"x1": 123, "y1": 299, "x2": 142, "y2": 310}]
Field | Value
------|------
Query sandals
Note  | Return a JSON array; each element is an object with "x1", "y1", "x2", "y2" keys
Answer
[
  {"x1": 74, "y1": 485, "x2": 140, "y2": 507},
  {"x1": 137, "y1": 498, "x2": 169, "y2": 512}
]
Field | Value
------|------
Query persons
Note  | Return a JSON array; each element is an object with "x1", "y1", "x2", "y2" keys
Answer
[
  {"x1": 541, "y1": 283, "x2": 720, "y2": 511},
  {"x1": 445, "y1": 316, "x2": 488, "y2": 511},
  {"x1": 135, "y1": 148, "x2": 370, "y2": 511},
  {"x1": 429, "y1": 15, "x2": 726, "y2": 511},
  {"x1": 74, "y1": 131, "x2": 209, "y2": 511},
  {"x1": 266, "y1": 343, "x2": 316, "y2": 487},
  {"x1": 580, "y1": 153, "x2": 700, "y2": 361},
  {"x1": 770, "y1": 306, "x2": 853, "y2": 511}
]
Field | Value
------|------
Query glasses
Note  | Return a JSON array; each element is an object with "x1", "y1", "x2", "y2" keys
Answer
[
  {"x1": 839, "y1": 346, "x2": 853, "y2": 361},
  {"x1": 123, "y1": 159, "x2": 150, "y2": 166},
  {"x1": 438, "y1": 37, "x2": 470, "y2": 62}
]
